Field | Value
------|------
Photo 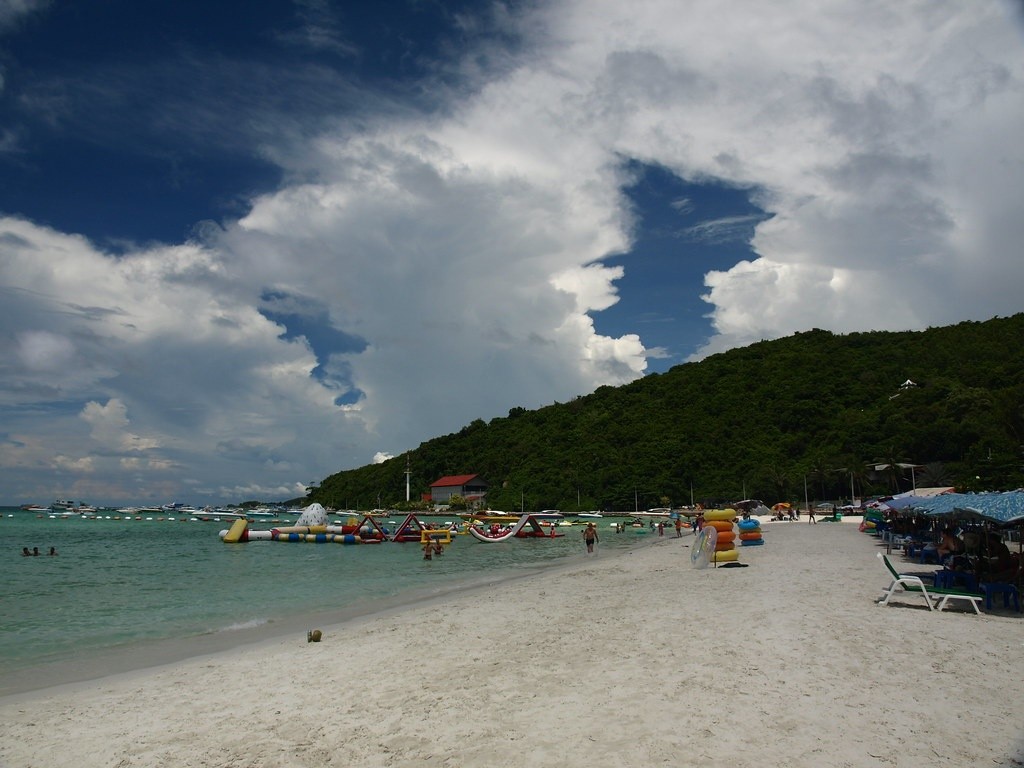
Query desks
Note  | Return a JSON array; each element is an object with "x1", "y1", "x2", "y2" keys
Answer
[
  {"x1": 920, "y1": 549, "x2": 938, "y2": 563},
  {"x1": 979, "y1": 581, "x2": 1019, "y2": 612},
  {"x1": 934, "y1": 570, "x2": 974, "y2": 592},
  {"x1": 908, "y1": 544, "x2": 922, "y2": 558},
  {"x1": 875, "y1": 524, "x2": 889, "y2": 535}
]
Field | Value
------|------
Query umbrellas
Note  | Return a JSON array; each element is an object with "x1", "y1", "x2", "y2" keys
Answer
[{"x1": 863, "y1": 489, "x2": 1024, "y2": 613}]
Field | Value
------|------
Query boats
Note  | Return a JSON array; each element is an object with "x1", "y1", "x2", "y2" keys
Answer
[
  {"x1": 20, "y1": 499, "x2": 390, "y2": 519},
  {"x1": 459, "y1": 510, "x2": 604, "y2": 520}
]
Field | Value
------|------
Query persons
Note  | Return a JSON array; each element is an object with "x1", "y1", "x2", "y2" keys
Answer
[
  {"x1": 809, "y1": 504, "x2": 817, "y2": 525},
  {"x1": 615, "y1": 507, "x2": 802, "y2": 538},
  {"x1": 32, "y1": 547, "x2": 42, "y2": 556},
  {"x1": 933, "y1": 528, "x2": 966, "y2": 565},
  {"x1": 21, "y1": 547, "x2": 32, "y2": 556},
  {"x1": 982, "y1": 533, "x2": 1010, "y2": 572},
  {"x1": 47, "y1": 547, "x2": 59, "y2": 556},
  {"x1": 584, "y1": 523, "x2": 599, "y2": 553},
  {"x1": 832, "y1": 505, "x2": 839, "y2": 519},
  {"x1": 380, "y1": 523, "x2": 556, "y2": 561},
  {"x1": 581, "y1": 530, "x2": 584, "y2": 533}
]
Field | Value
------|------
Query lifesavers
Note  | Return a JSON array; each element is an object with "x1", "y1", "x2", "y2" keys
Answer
[
  {"x1": 737, "y1": 519, "x2": 764, "y2": 546},
  {"x1": 703, "y1": 509, "x2": 738, "y2": 561},
  {"x1": 691, "y1": 526, "x2": 717, "y2": 569}
]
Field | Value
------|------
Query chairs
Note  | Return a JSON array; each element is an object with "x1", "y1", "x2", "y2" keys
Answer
[{"x1": 770, "y1": 500, "x2": 1024, "y2": 615}]
